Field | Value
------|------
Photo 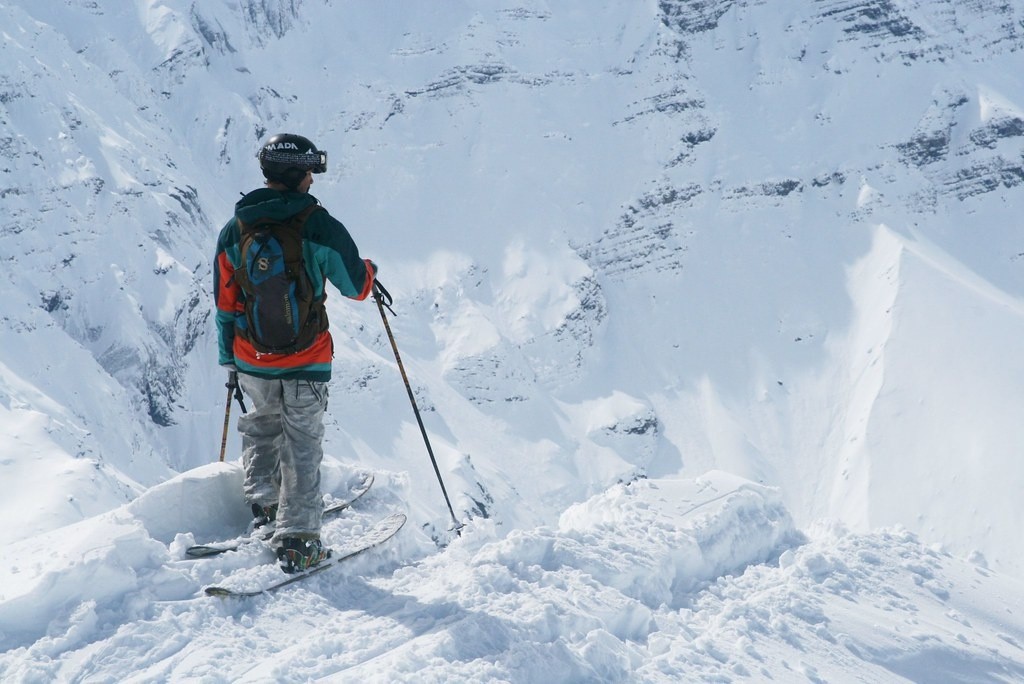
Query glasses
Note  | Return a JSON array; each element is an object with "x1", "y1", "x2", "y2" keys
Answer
[{"x1": 320, "y1": 151, "x2": 327, "y2": 166}]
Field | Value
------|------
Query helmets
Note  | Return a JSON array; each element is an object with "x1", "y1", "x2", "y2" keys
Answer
[{"x1": 259, "y1": 133, "x2": 327, "y2": 187}]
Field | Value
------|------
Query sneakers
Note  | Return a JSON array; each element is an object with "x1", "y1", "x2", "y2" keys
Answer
[
  {"x1": 277, "y1": 537, "x2": 333, "y2": 574},
  {"x1": 251, "y1": 503, "x2": 279, "y2": 541}
]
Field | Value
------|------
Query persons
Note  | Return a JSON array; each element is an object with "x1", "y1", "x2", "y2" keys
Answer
[{"x1": 214, "y1": 133, "x2": 378, "y2": 574}]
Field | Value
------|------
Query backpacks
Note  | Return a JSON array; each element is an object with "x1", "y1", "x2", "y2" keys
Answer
[{"x1": 240, "y1": 223, "x2": 318, "y2": 356}]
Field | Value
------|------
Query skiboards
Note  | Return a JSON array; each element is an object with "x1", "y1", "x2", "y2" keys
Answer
[{"x1": 185, "y1": 473, "x2": 408, "y2": 597}]
